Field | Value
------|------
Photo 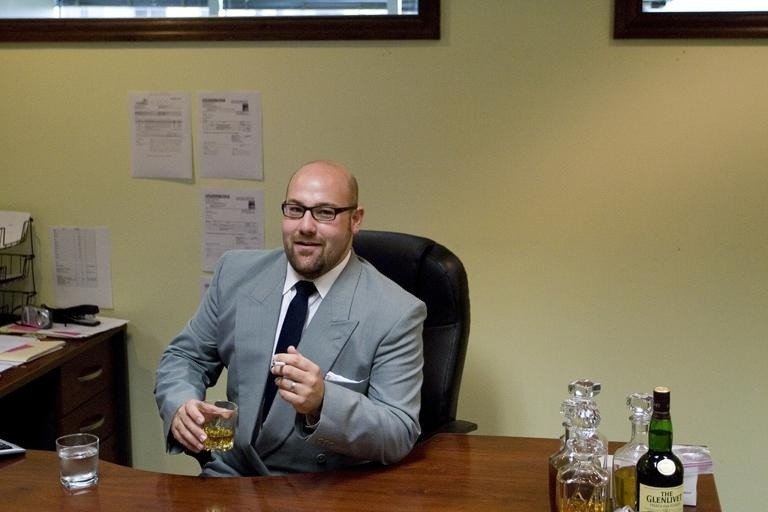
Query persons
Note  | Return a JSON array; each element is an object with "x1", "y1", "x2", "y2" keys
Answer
[{"x1": 154, "y1": 159, "x2": 427, "y2": 477}]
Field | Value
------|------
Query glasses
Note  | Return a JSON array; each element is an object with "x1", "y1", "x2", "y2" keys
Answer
[{"x1": 280, "y1": 202, "x2": 356, "y2": 222}]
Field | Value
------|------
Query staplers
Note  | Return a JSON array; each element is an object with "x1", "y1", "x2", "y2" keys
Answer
[{"x1": 52, "y1": 304, "x2": 100, "y2": 327}]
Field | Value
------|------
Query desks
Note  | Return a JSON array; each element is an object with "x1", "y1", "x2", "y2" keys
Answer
[{"x1": 0, "y1": 315, "x2": 722, "y2": 511}]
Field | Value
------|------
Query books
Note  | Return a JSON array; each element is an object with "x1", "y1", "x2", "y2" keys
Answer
[{"x1": 0, "y1": 335, "x2": 66, "y2": 364}]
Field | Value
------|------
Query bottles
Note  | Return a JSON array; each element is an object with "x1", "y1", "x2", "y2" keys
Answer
[{"x1": 548, "y1": 380, "x2": 684, "y2": 511}]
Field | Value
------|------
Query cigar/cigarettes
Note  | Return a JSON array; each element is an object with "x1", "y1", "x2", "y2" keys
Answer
[{"x1": 272, "y1": 361, "x2": 286, "y2": 367}]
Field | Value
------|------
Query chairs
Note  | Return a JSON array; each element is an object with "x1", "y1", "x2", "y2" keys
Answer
[{"x1": 349, "y1": 229, "x2": 478, "y2": 439}]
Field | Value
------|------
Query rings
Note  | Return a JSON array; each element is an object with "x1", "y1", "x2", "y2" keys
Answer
[{"x1": 290, "y1": 382, "x2": 297, "y2": 391}]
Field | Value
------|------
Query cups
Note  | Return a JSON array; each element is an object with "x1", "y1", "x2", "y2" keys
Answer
[
  {"x1": 55, "y1": 433, "x2": 100, "y2": 495},
  {"x1": 198, "y1": 401, "x2": 239, "y2": 452}
]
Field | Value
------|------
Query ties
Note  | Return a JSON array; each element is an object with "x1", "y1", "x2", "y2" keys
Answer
[{"x1": 262, "y1": 281, "x2": 319, "y2": 426}]
